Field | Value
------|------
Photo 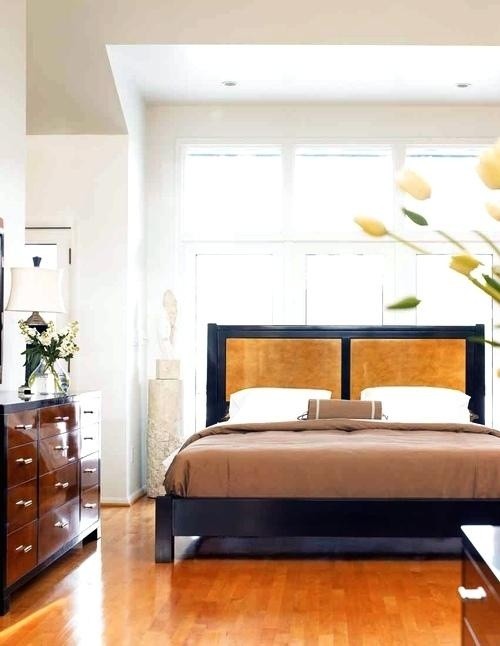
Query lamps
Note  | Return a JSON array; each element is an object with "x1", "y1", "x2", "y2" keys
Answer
[{"x1": 7, "y1": 256, "x2": 66, "y2": 324}]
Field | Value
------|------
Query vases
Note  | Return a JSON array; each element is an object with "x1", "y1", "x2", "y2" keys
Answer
[{"x1": 28, "y1": 355, "x2": 70, "y2": 399}]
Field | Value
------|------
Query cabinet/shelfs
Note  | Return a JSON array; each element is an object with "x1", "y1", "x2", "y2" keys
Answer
[
  {"x1": 455, "y1": 525, "x2": 500, "y2": 645},
  {"x1": 1, "y1": 391, "x2": 102, "y2": 616}
]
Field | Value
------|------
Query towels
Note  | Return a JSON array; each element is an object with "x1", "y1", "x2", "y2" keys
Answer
[{"x1": 307, "y1": 398, "x2": 383, "y2": 419}]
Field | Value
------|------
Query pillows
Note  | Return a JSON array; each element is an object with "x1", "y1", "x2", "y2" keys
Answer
[
  {"x1": 229, "y1": 387, "x2": 332, "y2": 422},
  {"x1": 360, "y1": 386, "x2": 472, "y2": 424}
]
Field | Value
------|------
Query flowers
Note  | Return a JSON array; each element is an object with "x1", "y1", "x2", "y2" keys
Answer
[
  {"x1": 18, "y1": 320, "x2": 80, "y2": 395},
  {"x1": 351, "y1": 142, "x2": 500, "y2": 377}
]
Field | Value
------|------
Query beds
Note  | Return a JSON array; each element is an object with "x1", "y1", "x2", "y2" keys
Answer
[{"x1": 155, "y1": 323, "x2": 500, "y2": 563}]
